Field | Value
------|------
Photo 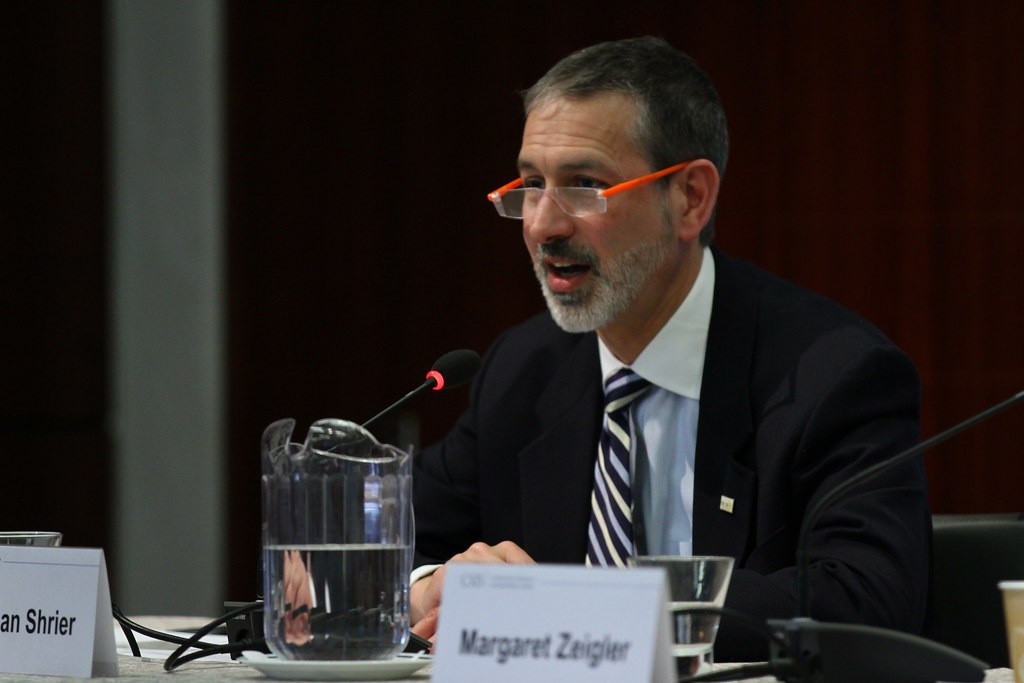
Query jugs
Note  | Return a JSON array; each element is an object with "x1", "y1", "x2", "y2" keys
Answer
[{"x1": 261, "y1": 418, "x2": 411, "y2": 658}]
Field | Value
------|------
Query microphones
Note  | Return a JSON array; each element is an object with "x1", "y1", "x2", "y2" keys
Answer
[{"x1": 223, "y1": 350, "x2": 483, "y2": 659}]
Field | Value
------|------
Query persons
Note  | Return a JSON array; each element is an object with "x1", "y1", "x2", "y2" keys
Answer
[{"x1": 284, "y1": 36, "x2": 933, "y2": 683}]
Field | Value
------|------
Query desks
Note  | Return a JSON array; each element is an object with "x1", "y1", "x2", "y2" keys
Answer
[{"x1": 1, "y1": 615, "x2": 1017, "y2": 683}]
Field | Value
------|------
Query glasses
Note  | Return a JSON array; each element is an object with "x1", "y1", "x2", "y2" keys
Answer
[{"x1": 487, "y1": 159, "x2": 695, "y2": 220}]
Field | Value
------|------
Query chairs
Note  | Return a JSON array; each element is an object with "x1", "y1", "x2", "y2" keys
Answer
[{"x1": 924, "y1": 514, "x2": 1024, "y2": 671}]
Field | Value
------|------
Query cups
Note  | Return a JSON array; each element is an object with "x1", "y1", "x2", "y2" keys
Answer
[
  {"x1": 1, "y1": 531, "x2": 61, "y2": 548},
  {"x1": 625, "y1": 557, "x2": 735, "y2": 671}
]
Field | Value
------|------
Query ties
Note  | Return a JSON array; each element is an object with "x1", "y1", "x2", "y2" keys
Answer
[{"x1": 585, "y1": 369, "x2": 650, "y2": 567}]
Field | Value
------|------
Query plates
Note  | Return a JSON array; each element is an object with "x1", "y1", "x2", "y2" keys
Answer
[{"x1": 243, "y1": 651, "x2": 433, "y2": 680}]
